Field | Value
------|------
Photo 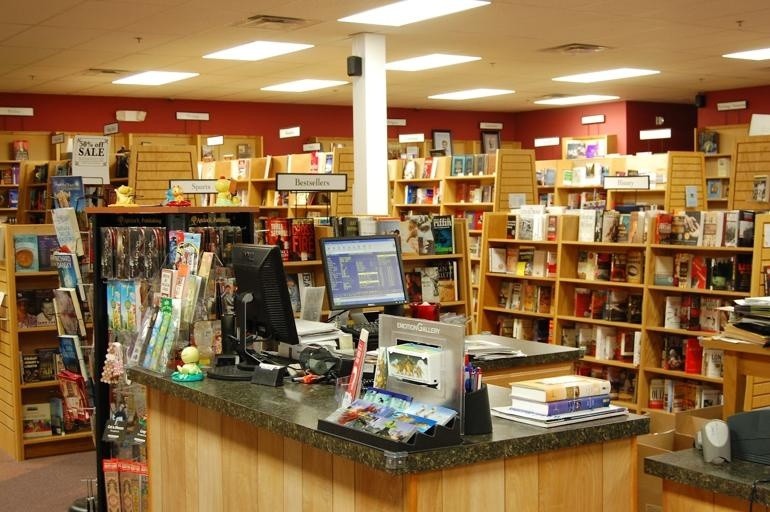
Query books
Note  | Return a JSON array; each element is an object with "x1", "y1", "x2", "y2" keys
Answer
[
  {"x1": 386, "y1": 146, "x2": 497, "y2": 334},
  {"x1": 577, "y1": 211, "x2": 649, "y2": 284},
  {"x1": 561, "y1": 287, "x2": 642, "y2": 365},
  {"x1": 253, "y1": 214, "x2": 458, "y2": 310},
  {"x1": 496, "y1": 282, "x2": 555, "y2": 345},
  {"x1": 660, "y1": 295, "x2": 733, "y2": 379},
  {"x1": 490, "y1": 362, "x2": 638, "y2": 428},
  {"x1": 704, "y1": 157, "x2": 730, "y2": 201},
  {"x1": 753, "y1": 176, "x2": 768, "y2": 202},
  {"x1": 535, "y1": 158, "x2": 667, "y2": 209},
  {"x1": 1, "y1": 134, "x2": 110, "y2": 439},
  {"x1": 199, "y1": 153, "x2": 333, "y2": 206},
  {"x1": 476, "y1": 204, "x2": 555, "y2": 277},
  {"x1": 648, "y1": 378, "x2": 724, "y2": 412},
  {"x1": 714, "y1": 296, "x2": 770, "y2": 345},
  {"x1": 653, "y1": 210, "x2": 756, "y2": 292}
]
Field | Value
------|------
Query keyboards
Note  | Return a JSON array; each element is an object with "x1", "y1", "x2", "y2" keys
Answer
[{"x1": 339, "y1": 322, "x2": 381, "y2": 346}]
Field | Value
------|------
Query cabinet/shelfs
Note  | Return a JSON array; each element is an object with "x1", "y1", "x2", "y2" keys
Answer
[
  {"x1": 694, "y1": 123, "x2": 749, "y2": 211},
  {"x1": 535, "y1": 150, "x2": 709, "y2": 211},
  {"x1": 476, "y1": 211, "x2": 770, "y2": 421},
  {"x1": 253, "y1": 217, "x2": 477, "y2": 334},
  {"x1": 388, "y1": 148, "x2": 539, "y2": 334}
]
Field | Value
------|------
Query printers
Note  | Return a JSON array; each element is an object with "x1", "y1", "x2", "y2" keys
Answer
[{"x1": 254, "y1": 319, "x2": 353, "y2": 362}]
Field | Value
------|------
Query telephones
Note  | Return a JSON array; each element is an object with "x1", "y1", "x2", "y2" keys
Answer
[{"x1": 215, "y1": 314, "x2": 239, "y2": 365}]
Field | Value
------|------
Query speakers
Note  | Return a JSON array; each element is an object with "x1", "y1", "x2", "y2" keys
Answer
[
  {"x1": 695, "y1": 95, "x2": 704, "y2": 108},
  {"x1": 346, "y1": 56, "x2": 363, "y2": 77}
]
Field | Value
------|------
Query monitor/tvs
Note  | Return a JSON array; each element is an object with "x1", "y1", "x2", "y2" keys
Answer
[
  {"x1": 206, "y1": 243, "x2": 299, "y2": 383},
  {"x1": 319, "y1": 234, "x2": 408, "y2": 323}
]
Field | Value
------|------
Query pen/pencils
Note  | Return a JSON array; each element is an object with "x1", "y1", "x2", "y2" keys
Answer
[
  {"x1": 292, "y1": 375, "x2": 326, "y2": 384},
  {"x1": 464, "y1": 353, "x2": 482, "y2": 393}
]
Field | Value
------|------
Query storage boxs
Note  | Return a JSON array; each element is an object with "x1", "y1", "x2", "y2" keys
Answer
[{"x1": 636, "y1": 404, "x2": 724, "y2": 512}]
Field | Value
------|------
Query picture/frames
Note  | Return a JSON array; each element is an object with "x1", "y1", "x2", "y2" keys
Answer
[
  {"x1": 480, "y1": 130, "x2": 500, "y2": 154},
  {"x1": 432, "y1": 129, "x2": 453, "y2": 155}
]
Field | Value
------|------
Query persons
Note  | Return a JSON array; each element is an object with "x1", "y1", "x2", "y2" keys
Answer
[{"x1": 442, "y1": 141, "x2": 448, "y2": 155}]
